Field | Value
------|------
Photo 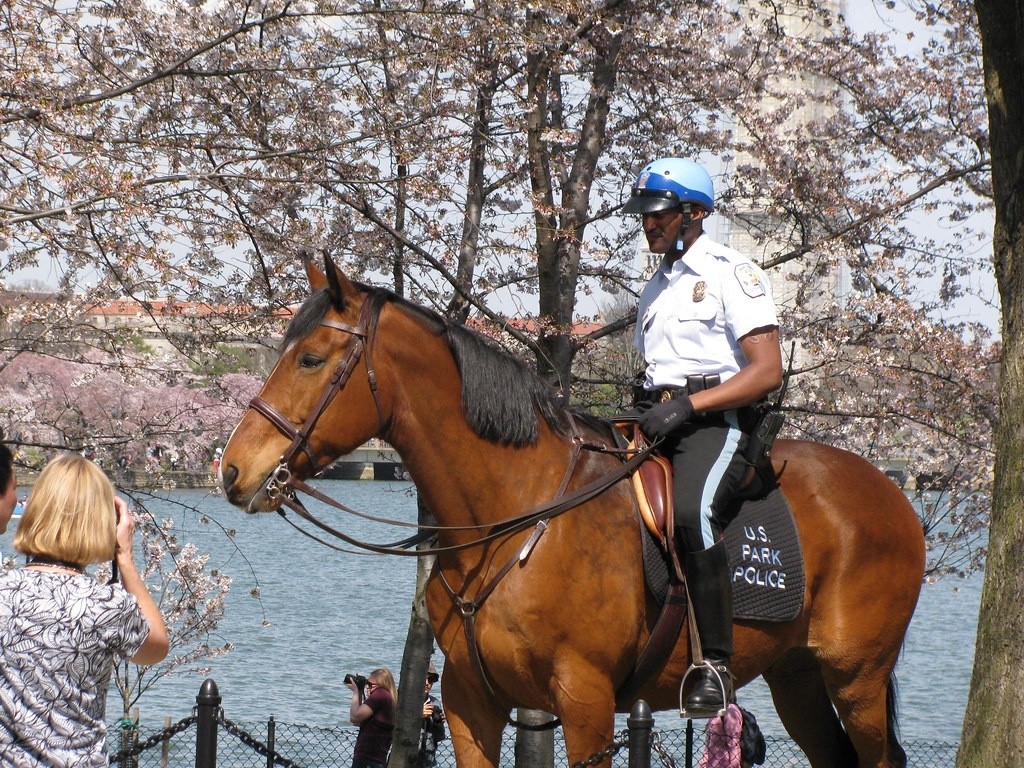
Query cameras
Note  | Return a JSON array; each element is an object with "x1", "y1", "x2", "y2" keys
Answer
[
  {"x1": 431, "y1": 705, "x2": 443, "y2": 722},
  {"x1": 344, "y1": 674, "x2": 366, "y2": 690}
]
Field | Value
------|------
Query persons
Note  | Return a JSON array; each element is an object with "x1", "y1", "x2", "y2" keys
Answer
[
  {"x1": 0, "y1": 446, "x2": 18, "y2": 535},
  {"x1": 415, "y1": 661, "x2": 447, "y2": 768},
  {"x1": 345, "y1": 668, "x2": 398, "y2": 768},
  {"x1": 0, "y1": 456, "x2": 169, "y2": 768},
  {"x1": 699, "y1": 698, "x2": 743, "y2": 768},
  {"x1": 630, "y1": 157, "x2": 782, "y2": 715}
]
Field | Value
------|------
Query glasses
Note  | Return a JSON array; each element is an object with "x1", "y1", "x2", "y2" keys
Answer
[
  {"x1": 366, "y1": 682, "x2": 379, "y2": 689},
  {"x1": 428, "y1": 676, "x2": 433, "y2": 684}
]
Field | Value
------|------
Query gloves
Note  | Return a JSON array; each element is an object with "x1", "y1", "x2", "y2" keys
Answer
[{"x1": 638, "y1": 392, "x2": 695, "y2": 441}]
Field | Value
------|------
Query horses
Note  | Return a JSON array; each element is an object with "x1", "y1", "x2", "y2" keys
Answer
[{"x1": 216, "y1": 249, "x2": 930, "y2": 768}]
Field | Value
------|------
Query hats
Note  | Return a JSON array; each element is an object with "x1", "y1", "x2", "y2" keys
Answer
[{"x1": 428, "y1": 660, "x2": 439, "y2": 677}]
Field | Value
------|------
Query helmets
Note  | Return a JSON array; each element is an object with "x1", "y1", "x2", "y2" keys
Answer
[{"x1": 622, "y1": 158, "x2": 714, "y2": 214}]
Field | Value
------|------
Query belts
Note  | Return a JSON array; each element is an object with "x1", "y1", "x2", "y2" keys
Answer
[{"x1": 637, "y1": 383, "x2": 687, "y2": 403}]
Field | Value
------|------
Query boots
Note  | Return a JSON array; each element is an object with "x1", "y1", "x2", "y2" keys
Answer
[{"x1": 684, "y1": 536, "x2": 737, "y2": 714}]
